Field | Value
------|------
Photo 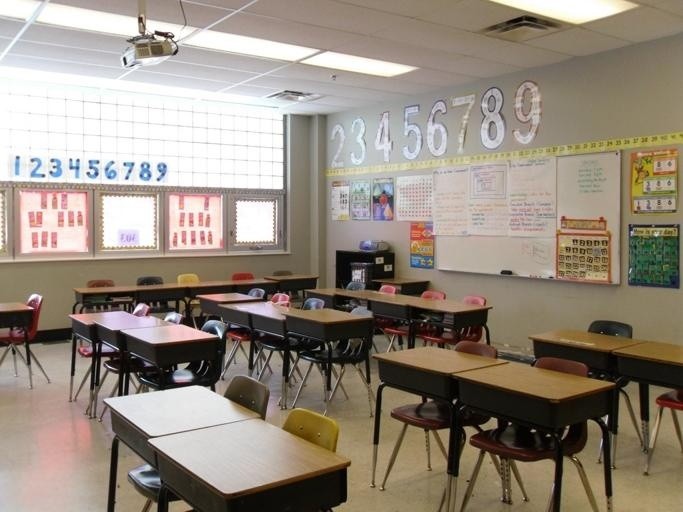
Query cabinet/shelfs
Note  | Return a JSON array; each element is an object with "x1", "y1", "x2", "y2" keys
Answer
[{"x1": 332, "y1": 246, "x2": 396, "y2": 304}]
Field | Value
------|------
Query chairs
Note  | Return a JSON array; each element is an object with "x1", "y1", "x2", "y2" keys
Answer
[
  {"x1": 64, "y1": 269, "x2": 681, "y2": 512},
  {"x1": 0, "y1": 293, "x2": 51, "y2": 385}
]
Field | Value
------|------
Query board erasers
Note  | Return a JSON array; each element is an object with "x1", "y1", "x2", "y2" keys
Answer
[{"x1": 501, "y1": 270, "x2": 513, "y2": 275}]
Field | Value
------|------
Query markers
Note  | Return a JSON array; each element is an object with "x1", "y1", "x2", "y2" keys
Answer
[{"x1": 542, "y1": 276, "x2": 553, "y2": 279}]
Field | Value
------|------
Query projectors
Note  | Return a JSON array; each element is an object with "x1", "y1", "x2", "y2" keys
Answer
[{"x1": 120, "y1": 40, "x2": 173, "y2": 67}]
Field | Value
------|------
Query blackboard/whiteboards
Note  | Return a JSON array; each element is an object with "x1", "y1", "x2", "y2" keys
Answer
[{"x1": 433, "y1": 152, "x2": 622, "y2": 285}]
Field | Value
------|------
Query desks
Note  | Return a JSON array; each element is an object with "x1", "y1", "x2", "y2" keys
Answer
[{"x1": 0, "y1": 301, "x2": 36, "y2": 390}]
